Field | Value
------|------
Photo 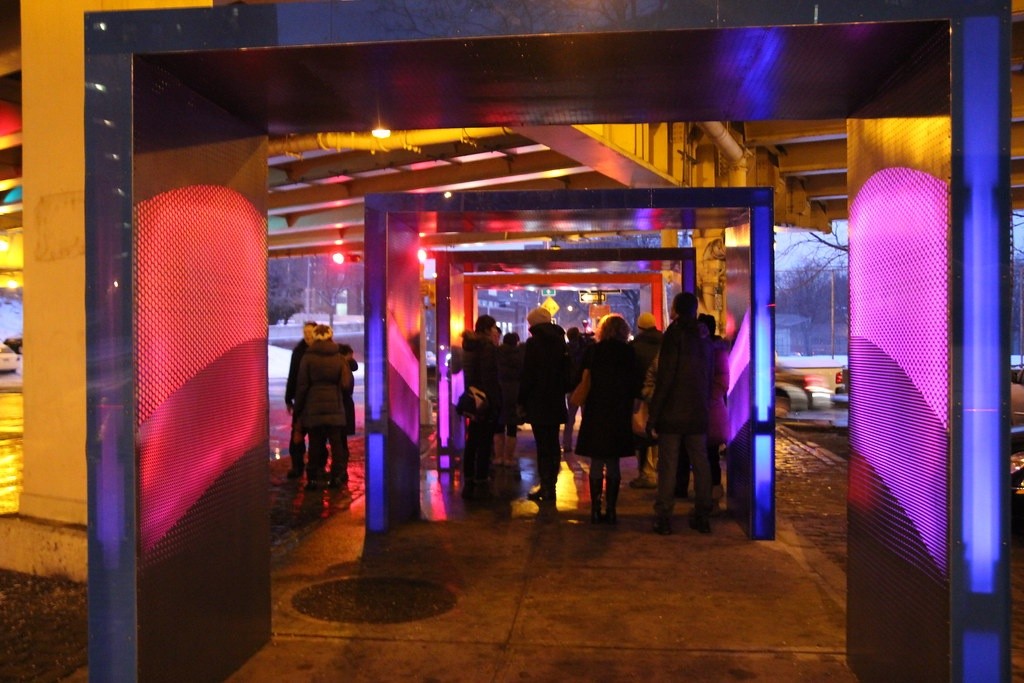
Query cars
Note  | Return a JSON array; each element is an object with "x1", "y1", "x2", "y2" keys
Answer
[
  {"x1": 1011, "y1": 368, "x2": 1024, "y2": 501},
  {"x1": 0, "y1": 341, "x2": 20, "y2": 374}
]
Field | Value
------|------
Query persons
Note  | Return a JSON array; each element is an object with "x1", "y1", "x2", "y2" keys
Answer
[
  {"x1": 285, "y1": 322, "x2": 358, "y2": 488},
  {"x1": 462, "y1": 292, "x2": 731, "y2": 535}
]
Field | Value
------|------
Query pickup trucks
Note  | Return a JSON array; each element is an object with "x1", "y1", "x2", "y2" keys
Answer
[{"x1": 775, "y1": 350, "x2": 848, "y2": 421}]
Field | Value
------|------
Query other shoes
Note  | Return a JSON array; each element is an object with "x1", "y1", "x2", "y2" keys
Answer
[
  {"x1": 653, "y1": 522, "x2": 673, "y2": 534},
  {"x1": 527, "y1": 487, "x2": 555, "y2": 498},
  {"x1": 629, "y1": 476, "x2": 657, "y2": 488},
  {"x1": 461, "y1": 481, "x2": 499, "y2": 499},
  {"x1": 689, "y1": 518, "x2": 710, "y2": 533}
]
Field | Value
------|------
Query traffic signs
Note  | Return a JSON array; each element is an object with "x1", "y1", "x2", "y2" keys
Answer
[{"x1": 579, "y1": 292, "x2": 606, "y2": 303}]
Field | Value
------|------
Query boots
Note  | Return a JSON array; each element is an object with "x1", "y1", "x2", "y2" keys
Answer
[
  {"x1": 605, "y1": 477, "x2": 619, "y2": 523},
  {"x1": 708, "y1": 484, "x2": 722, "y2": 515},
  {"x1": 492, "y1": 435, "x2": 505, "y2": 466},
  {"x1": 503, "y1": 435, "x2": 517, "y2": 467},
  {"x1": 589, "y1": 476, "x2": 603, "y2": 524}
]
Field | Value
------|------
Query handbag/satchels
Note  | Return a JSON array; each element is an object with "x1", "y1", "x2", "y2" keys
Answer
[{"x1": 456, "y1": 381, "x2": 495, "y2": 427}]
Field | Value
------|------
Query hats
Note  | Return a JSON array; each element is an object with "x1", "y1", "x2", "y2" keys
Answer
[
  {"x1": 527, "y1": 307, "x2": 551, "y2": 326},
  {"x1": 638, "y1": 311, "x2": 656, "y2": 331},
  {"x1": 313, "y1": 324, "x2": 333, "y2": 342}
]
